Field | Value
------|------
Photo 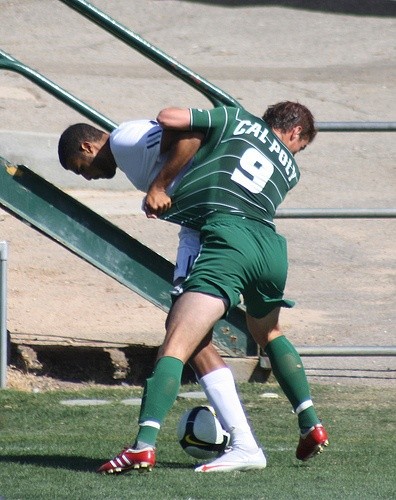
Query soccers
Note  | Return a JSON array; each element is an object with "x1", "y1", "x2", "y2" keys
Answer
[{"x1": 177, "y1": 404, "x2": 230, "y2": 459}]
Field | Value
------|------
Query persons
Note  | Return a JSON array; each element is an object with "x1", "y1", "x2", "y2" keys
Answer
[
  {"x1": 58, "y1": 120, "x2": 267, "y2": 474},
  {"x1": 95, "y1": 102, "x2": 329, "y2": 475}
]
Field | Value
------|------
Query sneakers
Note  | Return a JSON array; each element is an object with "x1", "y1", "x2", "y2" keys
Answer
[
  {"x1": 195, "y1": 447, "x2": 267, "y2": 473},
  {"x1": 295, "y1": 427, "x2": 328, "y2": 462},
  {"x1": 96, "y1": 447, "x2": 156, "y2": 474}
]
